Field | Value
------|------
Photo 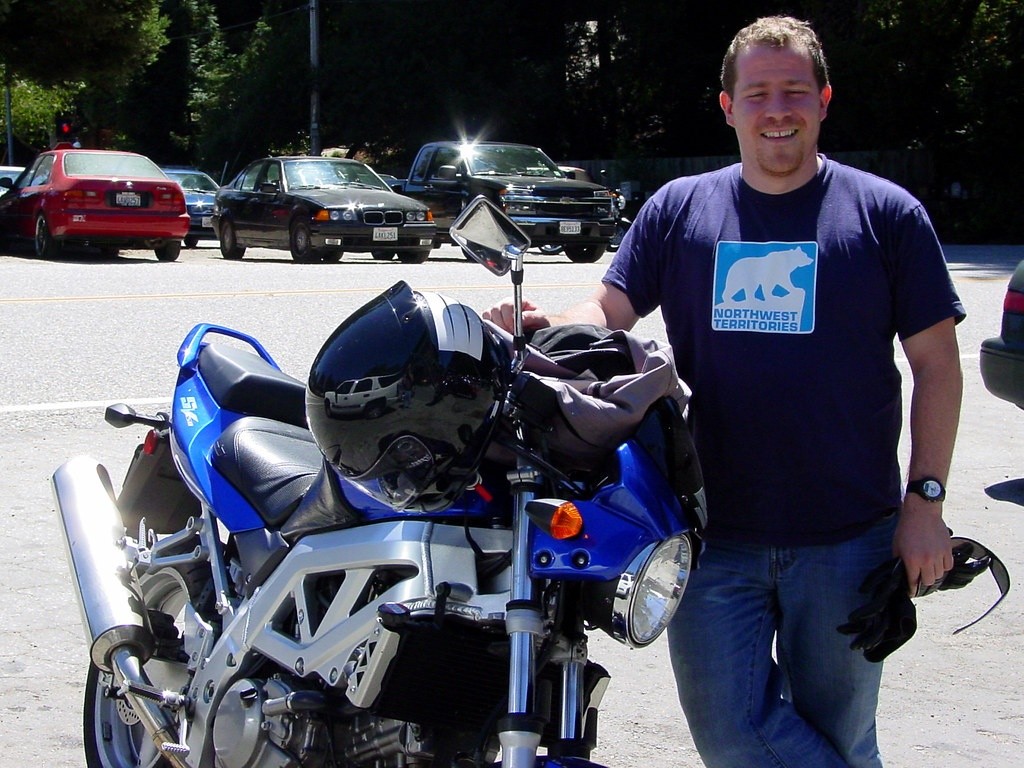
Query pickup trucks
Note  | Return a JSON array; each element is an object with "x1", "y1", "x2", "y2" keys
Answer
[{"x1": 385, "y1": 141, "x2": 620, "y2": 263}]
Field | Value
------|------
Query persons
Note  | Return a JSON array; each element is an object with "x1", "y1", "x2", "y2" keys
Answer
[{"x1": 483, "y1": 15, "x2": 968, "y2": 768}]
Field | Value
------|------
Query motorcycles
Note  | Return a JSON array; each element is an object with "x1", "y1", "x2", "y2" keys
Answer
[
  {"x1": 538, "y1": 169, "x2": 632, "y2": 255},
  {"x1": 52, "y1": 193, "x2": 710, "y2": 768}
]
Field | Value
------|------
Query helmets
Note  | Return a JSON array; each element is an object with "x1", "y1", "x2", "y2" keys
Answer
[{"x1": 305, "y1": 280, "x2": 508, "y2": 514}]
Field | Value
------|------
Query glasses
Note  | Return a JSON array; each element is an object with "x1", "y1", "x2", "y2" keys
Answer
[{"x1": 892, "y1": 535, "x2": 1010, "y2": 636}]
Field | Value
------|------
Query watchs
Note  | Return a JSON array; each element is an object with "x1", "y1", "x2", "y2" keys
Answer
[{"x1": 906, "y1": 475, "x2": 946, "y2": 501}]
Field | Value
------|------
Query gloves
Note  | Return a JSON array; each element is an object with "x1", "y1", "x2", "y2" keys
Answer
[{"x1": 836, "y1": 526, "x2": 990, "y2": 663}]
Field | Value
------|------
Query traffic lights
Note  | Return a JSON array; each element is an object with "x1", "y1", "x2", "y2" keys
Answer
[{"x1": 54, "y1": 110, "x2": 71, "y2": 141}]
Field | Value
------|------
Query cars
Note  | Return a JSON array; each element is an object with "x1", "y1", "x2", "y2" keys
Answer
[
  {"x1": 0, "y1": 149, "x2": 191, "y2": 263},
  {"x1": 158, "y1": 168, "x2": 225, "y2": 248},
  {"x1": 214, "y1": 155, "x2": 437, "y2": 264},
  {"x1": 0, "y1": 166, "x2": 28, "y2": 198}
]
